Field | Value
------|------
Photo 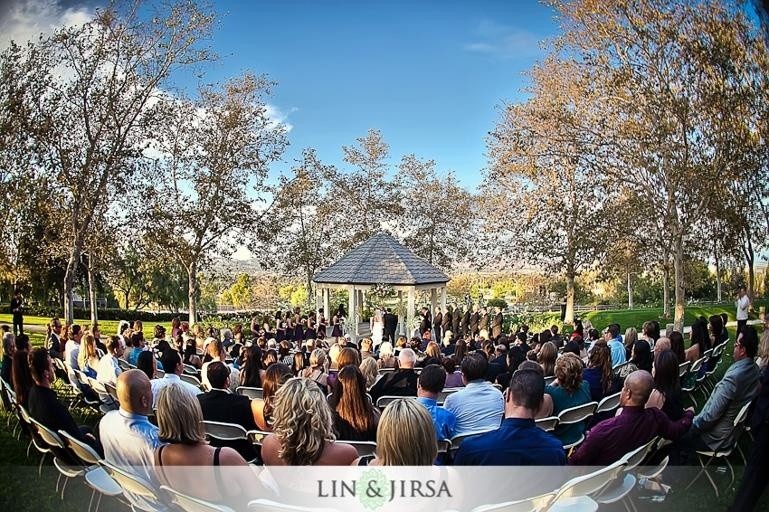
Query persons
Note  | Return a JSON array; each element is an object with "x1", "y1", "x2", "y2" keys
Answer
[
  {"x1": 262, "y1": 315, "x2": 274, "y2": 340},
  {"x1": 28, "y1": 346, "x2": 104, "y2": 467},
  {"x1": 372, "y1": 309, "x2": 384, "y2": 351},
  {"x1": 455, "y1": 368, "x2": 569, "y2": 466},
  {"x1": 422, "y1": 307, "x2": 432, "y2": 337},
  {"x1": 121, "y1": 317, "x2": 245, "y2": 381},
  {"x1": 451, "y1": 302, "x2": 460, "y2": 338},
  {"x1": 275, "y1": 311, "x2": 285, "y2": 343},
  {"x1": 756, "y1": 329, "x2": 769, "y2": 370},
  {"x1": 590, "y1": 322, "x2": 662, "y2": 340},
  {"x1": 544, "y1": 351, "x2": 593, "y2": 443},
  {"x1": 317, "y1": 308, "x2": 329, "y2": 339},
  {"x1": 478, "y1": 307, "x2": 490, "y2": 340},
  {"x1": 720, "y1": 314, "x2": 729, "y2": 350},
  {"x1": 95, "y1": 336, "x2": 131, "y2": 410},
  {"x1": 305, "y1": 312, "x2": 316, "y2": 339},
  {"x1": 66, "y1": 323, "x2": 82, "y2": 369},
  {"x1": 519, "y1": 326, "x2": 529, "y2": 332},
  {"x1": 77, "y1": 333, "x2": 100, "y2": 376},
  {"x1": 415, "y1": 364, "x2": 456, "y2": 466},
  {"x1": 651, "y1": 350, "x2": 686, "y2": 420},
  {"x1": 332, "y1": 309, "x2": 343, "y2": 343},
  {"x1": 674, "y1": 325, "x2": 760, "y2": 452},
  {"x1": 294, "y1": 307, "x2": 306, "y2": 348},
  {"x1": 285, "y1": 311, "x2": 294, "y2": 344},
  {"x1": 614, "y1": 340, "x2": 653, "y2": 390},
  {"x1": 669, "y1": 331, "x2": 686, "y2": 365},
  {"x1": 689, "y1": 320, "x2": 712, "y2": 358},
  {"x1": 352, "y1": 398, "x2": 454, "y2": 511},
  {"x1": 733, "y1": 284, "x2": 750, "y2": 342},
  {"x1": 490, "y1": 306, "x2": 503, "y2": 339},
  {"x1": 247, "y1": 336, "x2": 416, "y2": 384},
  {"x1": 470, "y1": 305, "x2": 478, "y2": 336},
  {"x1": 0, "y1": 331, "x2": 15, "y2": 386},
  {"x1": 384, "y1": 308, "x2": 398, "y2": 348},
  {"x1": 417, "y1": 333, "x2": 542, "y2": 368},
  {"x1": 460, "y1": 305, "x2": 470, "y2": 336},
  {"x1": 98, "y1": 369, "x2": 169, "y2": 512},
  {"x1": 13, "y1": 334, "x2": 31, "y2": 403},
  {"x1": 49, "y1": 318, "x2": 64, "y2": 361},
  {"x1": 709, "y1": 315, "x2": 723, "y2": 372},
  {"x1": 652, "y1": 337, "x2": 671, "y2": 377},
  {"x1": 11, "y1": 288, "x2": 24, "y2": 336},
  {"x1": 443, "y1": 307, "x2": 452, "y2": 339},
  {"x1": 263, "y1": 379, "x2": 358, "y2": 509},
  {"x1": 154, "y1": 382, "x2": 261, "y2": 511},
  {"x1": 251, "y1": 313, "x2": 262, "y2": 339},
  {"x1": 544, "y1": 321, "x2": 589, "y2": 351},
  {"x1": 567, "y1": 370, "x2": 694, "y2": 466},
  {"x1": 585, "y1": 338, "x2": 615, "y2": 399},
  {"x1": 433, "y1": 307, "x2": 443, "y2": 343}
]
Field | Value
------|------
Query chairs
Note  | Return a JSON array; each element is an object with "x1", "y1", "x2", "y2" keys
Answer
[
  {"x1": 181, "y1": 374, "x2": 200, "y2": 386},
  {"x1": 99, "y1": 460, "x2": 161, "y2": 512},
  {"x1": 329, "y1": 440, "x2": 377, "y2": 455},
  {"x1": 558, "y1": 402, "x2": 598, "y2": 458},
  {"x1": 199, "y1": 420, "x2": 246, "y2": 458},
  {"x1": 118, "y1": 357, "x2": 137, "y2": 369},
  {"x1": 236, "y1": 385, "x2": 265, "y2": 400},
  {"x1": 636, "y1": 455, "x2": 671, "y2": 499},
  {"x1": 551, "y1": 461, "x2": 626, "y2": 511},
  {"x1": 0, "y1": 377, "x2": 18, "y2": 438},
  {"x1": 684, "y1": 401, "x2": 753, "y2": 499},
  {"x1": 377, "y1": 368, "x2": 396, "y2": 374},
  {"x1": 29, "y1": 416, "x2": 85, "y2": 500},
  {"x1": 448, "y1": 431, "x2": 490, "y2": 464},
  {"x1": 248, "y1": 499, "x2": 332, "y2": 512},
  {"x1": 58, "y1": 429, "x2": 123, "y2": 512},
  {"x1": 159, "y1": 485, "x2": 223, "y2": 512},
  {"x1": 595, "y1": 393, "x2": 622, "y2": 419},
  {"x1": 535, "y1": 417, "x2": 560, "y2": 433},
  {"x1": 183, "y1": 362, "x2": 197, "y2": 374},
  {"x1": 18, "y1": 404, "x2": 57, "y2": 478},
  {"x1": 376, "y1": 396, "x2": 418, "y2": 410},
  {"x1": 594, "y1": 434, "x2": 660, "y2": 511},
  {"x1": 474, "y1": 493, "x2": 555, "y2": 512},
  {"x1": 678, "y1": 338, "x2": 732, "y2": 407},
  {"x1": 246, "y1": 430, "x2": 274, "y2": 458},
  {"x1": 156, "y1": 368, "x2": 165, "y2": 377},
  {"x1": 327, "y1": 391, "x2": 373, "y2": 406},
  {"x1": 436, "y1": 438, "x2": 453, "y2": 466},
  {"x1": 54, "y1": 358, "x2": 121, "y2": 428}
]
